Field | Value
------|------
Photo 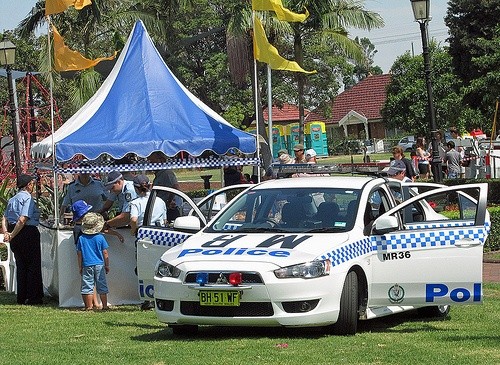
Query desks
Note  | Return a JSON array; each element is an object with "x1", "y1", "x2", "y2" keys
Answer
[{"x1": 39, "y1": 224, "x2": 142, "y2": 306}]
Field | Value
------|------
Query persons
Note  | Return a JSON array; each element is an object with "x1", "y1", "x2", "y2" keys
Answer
[
  {"x1": 2, "y1": 173, "x2": 46, "y2": 306},
  {"x1": 224, "y1": 144, "x2": 331, "y2": 221},
  {"x1": 59, "y1": 168, "x2": 182, "y2": 311},
  {"x1": 368, "y1": 127, "x2": 465, "y2": 209}
]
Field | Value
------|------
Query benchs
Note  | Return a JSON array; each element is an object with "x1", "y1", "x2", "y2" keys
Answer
[{"x1": 312, "y1": 200, "x2": 401, "y2": 222}]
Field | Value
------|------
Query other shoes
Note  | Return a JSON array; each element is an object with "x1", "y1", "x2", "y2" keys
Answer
[
  {"x1": 139, "y1": 300, "x2": 155, "y2": 309},
  {"x1": 18, "y1": 299, "x2": 44, "y2": 305}
]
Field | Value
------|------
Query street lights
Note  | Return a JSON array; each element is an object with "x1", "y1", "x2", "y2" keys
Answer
[
  {"x1": 0, "y1": 33, "x2": 22, "y2": 190},
  {"x1": 410, "y1": 0, "x2": 443, "y2": 183}
]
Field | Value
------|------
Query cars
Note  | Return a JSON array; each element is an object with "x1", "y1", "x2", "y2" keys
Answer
[
  {"x1": 329, "y1": 140, "x2": 367, "y2": 154},
  {"x1": 135, "y1": 174, "x2": 492, "y2": 336},
  {"x1": 397, "y1": 136, "x2": 418, "y2": 152}
]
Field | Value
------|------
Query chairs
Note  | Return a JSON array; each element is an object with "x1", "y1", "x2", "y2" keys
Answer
[
  {"x1": 0, "y1": 234, "x2": 11, "y2": 292},
  {"x1": 347, "y1": 200, "x2": 373, "y2": 220},
  {"x1": 282, "y1": 203, "x2": 306, "y2": 222}
]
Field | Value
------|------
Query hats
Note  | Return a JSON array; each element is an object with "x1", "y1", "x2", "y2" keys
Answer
[
  {"x1": 293, "y1": 144, "x2": 304, "y2": 151},
  {"x1": 276, "y1": 148, "x2": 290, "y2": 157},
  {"x1": 386, "y1": 160, "x2": 406, "y2": 175},
  {"x1": 305, "y1": 149, "x2": 317, "y2": 158},
  {"x1": 103, "y1": 170, "x2": 123, "y2": 190},
  {"x1": 278, "y1": 154, "x2": 296, "y2": 164},
  {"x1": 81, "y1": 212, "x2": 104, "y2": 235},
  {"x1": 16, "y1": 174, "x2": 32, "y2": 187},
  {"x1": 71, "y1": 199, "x2": 93, "y2": 222},
  {"x1": 131, "y1": 174, "x2": 150, "y2": 187}
]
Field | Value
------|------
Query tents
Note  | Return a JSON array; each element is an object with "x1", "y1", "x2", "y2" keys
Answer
[{"x1": 31, "y1": 19, "x2": 261, "y2": 224}]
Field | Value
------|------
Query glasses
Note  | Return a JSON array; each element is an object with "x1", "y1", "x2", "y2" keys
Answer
[{"x1": 391, "y1": 151, "x2": 396, "y2": 154}]
